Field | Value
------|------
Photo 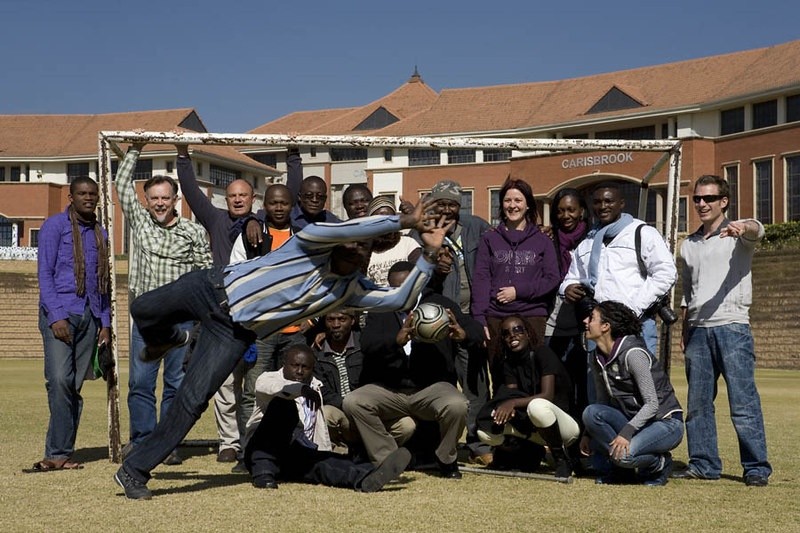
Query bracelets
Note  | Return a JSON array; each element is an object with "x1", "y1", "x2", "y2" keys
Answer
[{"x1": 420, "y1": 247, "x2": 441, "y2": 262}]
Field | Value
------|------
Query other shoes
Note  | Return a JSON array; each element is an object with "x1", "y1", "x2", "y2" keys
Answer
[
  {"x1": 644, "y1": 456, "x2": 673, "y2": 486},
  {"x1": 439, "y1": 460, "x2": 462, "y2": 479},
  {"x1": 113, "y1": 464, "x2": 152, "y2": 501},
  {"x1": 360, "y1": 447, "x2": 412, "y2": 493},
  {"x1": 746, "y1": 475, "x2": 768, "y2": 486},
  {"x1": 138, "y1": 330, "x2": 190, "y2": 364},
  {"x1": 670, "y1": 470, "x2": 695, "y2": 479},
  {"x1": 217, "y1": 448, "x2": 236, "y2": 462},
  {"x1": 22, "y1": 457, "x2": 79, "y2": 473},
  {"x1": 162, "y1": 448, "x2": 182, "y2": 466},
  {"x1": 595, "y1": 473, "x2": 629, "y2": 484},
  {"x1": 253, "y1": 473, "x2": 279, "y2": 489},
  {"x1": 468, "y1": 453, "x2": 493, "y2": 465}
]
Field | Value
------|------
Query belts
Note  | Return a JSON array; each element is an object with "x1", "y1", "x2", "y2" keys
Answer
[{"x1": 212, "y1": 287, "x2": 230, "y2": 315}]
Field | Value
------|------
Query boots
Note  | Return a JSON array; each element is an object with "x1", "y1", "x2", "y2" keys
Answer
[
  {"x1": 504, "y1": 434, "x2": 547, "y2": 473},
  {"x1": 535, "y1": 418, "x2": 574, "y2": 478}
]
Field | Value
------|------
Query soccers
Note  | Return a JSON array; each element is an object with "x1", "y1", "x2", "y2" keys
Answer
[{"x1": 408, "y1": 302, "x2": 452, "y2": 344}]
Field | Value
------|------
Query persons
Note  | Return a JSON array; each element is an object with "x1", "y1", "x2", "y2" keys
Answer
[
  {"x1": 398, "y1": 180, "x2": 494, "y2": 462},
  {"x1": 241, "y1": 176, "x2": 346, "y2": 347},
  {"x1": 340, "y1": 183, "x2": 415, "y2": 222},
  {"x1": 117, "y1": 127, "x2": 211, "y2": 465},
  {"x1": 169, "y1": 125, "x2": 303, "y2": 462},
  {"x1": 465, "y1": 313, "x2": 583, "y2": 473},
  {"x1": 113, "y1": 193, "x2": 457, "y2": 500},
  {"x1": 670, "y1": 175, "x2": 772, "y2": 486},
  {"x1": 580, "y1": 299, "x2": 684, "y2": 486},
  {"x1": 558, "y1": 181, "x2": 678, "y2": 406},
  {"x1": 32, "y1": 175, "x2": 109, "y2": 470},
  {"x1": 470, "y1": 172, "x2": 560, "y2": 442},
  {"x1": 540, "y1": 188, "x2": 597, "y2": 454},
  {"x1": 231, "y1": 183, "x2": 305, "y2": 471}
]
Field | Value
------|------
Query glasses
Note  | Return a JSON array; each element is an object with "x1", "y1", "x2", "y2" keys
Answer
[
  {"x1": 693, "y1": 195, "x2": 725, "y2": 203},
  {"x1": 299, "y1": 193, "x2": 326, "y2": 201},
  {"x1": 500, "y1": 325, "x2": 527, "y2": 337}
]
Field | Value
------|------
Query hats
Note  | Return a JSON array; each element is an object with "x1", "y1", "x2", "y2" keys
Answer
[{"x1": 431, "y1": 178, "x2": 462, "y2": 207}]
